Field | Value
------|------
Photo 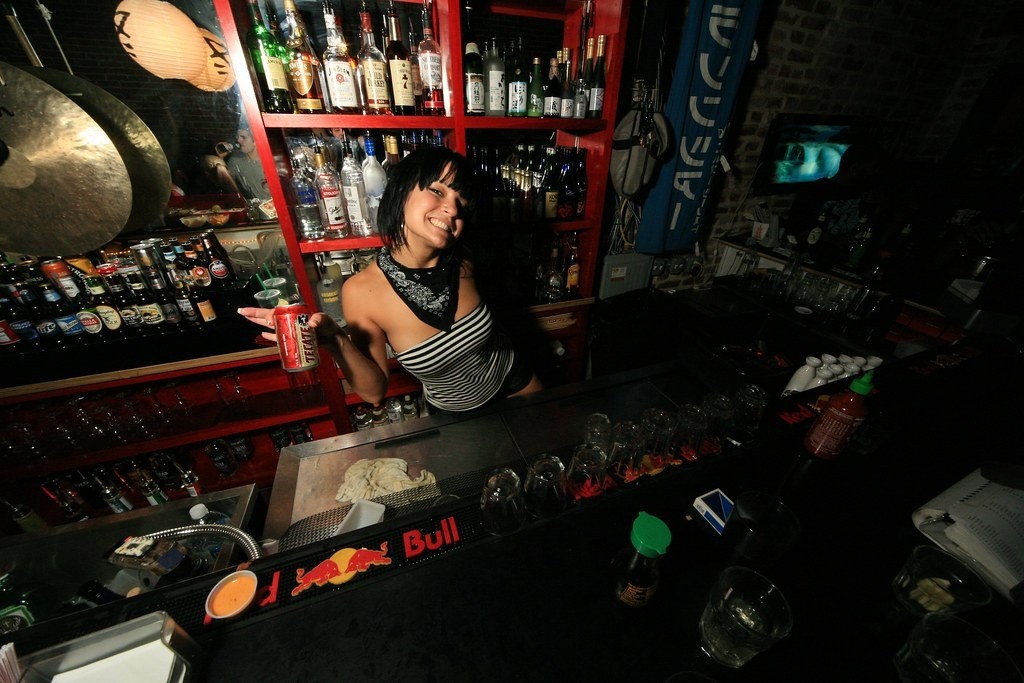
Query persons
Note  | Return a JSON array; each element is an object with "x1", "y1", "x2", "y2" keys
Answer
[
  {"x1": 222, "y1": 122, "x2": 273, "y2": 198},
  {"x1": 236, "y1": 144, "x2": 545, "y2": 420}
]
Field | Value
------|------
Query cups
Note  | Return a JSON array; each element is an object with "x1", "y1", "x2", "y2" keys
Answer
[
  {"x1": 893, "y1": 611, "x2": 1024, "y2": 683},
  {"x1": 765, "y1": 269, "x2": 855, "y2": 321},
  {"x1": 262, "y1": 274, "x2": 288, "y2": 305},
  {"x1": 253, "y1": 289, "x2": 282, "y2": 308},
  {"x1": 479, "y1": 384, "x2": 768, "y2": 538},
  {"x1": 706, "y1": 490, "x2": 802, "y2": 589}
]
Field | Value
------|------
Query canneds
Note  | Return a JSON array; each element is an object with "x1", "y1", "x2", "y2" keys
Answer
[
  {"x1": 272, "y1": 303, "x2": 321, "y2": 373},
  {"x1": 17, "y1": 238, "x2": 168, "y2": 301}
]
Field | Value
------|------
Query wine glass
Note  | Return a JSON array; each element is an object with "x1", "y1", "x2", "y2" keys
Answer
[
  {"x1": 861, "y1": 544, "x2": 993, "y2": 647},
  {"x1": 0, "y1": 372, "x2": 254, "y2": 464},
  {"x1": 666, "y1": 566, "x2": 793, "y2": 682}
]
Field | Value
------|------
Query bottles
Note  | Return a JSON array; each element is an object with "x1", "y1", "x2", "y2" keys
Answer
[
  {"x1": 79, "y1": 581, "x2": 126, "y2": 608},
  {"x1": 1, "y1": 421, "x2": 313, "y2": 532},
  {"x1": 0, "y1": 231, "x2": 237, "y2": 359},
  {"x1": 243, "y1": 0, "x2": 606, "y2": 332},
  {"x1": 188, "y1": 504, "x2": 236, "y2": 568},
  {"x1": 802, "y1": 205, "x2": 913, "y2": 268},
  {"x1": 782, "y1": 352, "x2": 884, "y2": 396},
  {"x1": 804, "y1": 369, "x2": 877, "y2": 461},
  {"x1": 349, "y1": 394, "x2": 428, "y2": 431}
]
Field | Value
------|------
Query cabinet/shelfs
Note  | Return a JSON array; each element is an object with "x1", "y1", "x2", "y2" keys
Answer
[{"x1": 0, "y1": 0, "x2": 630, "y2": 530}]
